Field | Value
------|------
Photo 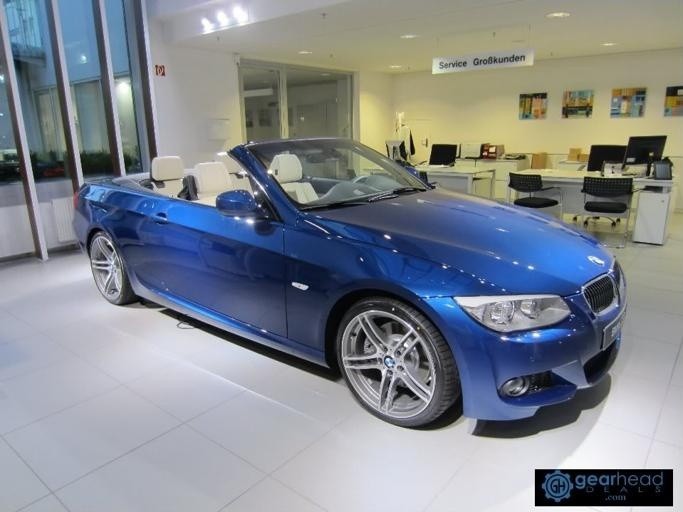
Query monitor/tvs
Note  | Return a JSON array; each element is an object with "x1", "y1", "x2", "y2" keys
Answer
[
  {"x1": 429, "y1": 144, "x2": 457, "y2": 167},
  {"x1": 587, "y1": 144, "x2": 627, "y2": 172},
  {"x1": 385, "y1": 140, "x2": 407, "y2": 161},
  {"x1": 626, "y1": 135, "x2": 667, "y2": 164}
]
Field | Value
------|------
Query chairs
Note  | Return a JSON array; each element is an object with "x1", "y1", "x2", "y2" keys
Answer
[
  {"x1": 268, "y1": 151, "x2": 319, "y2": 204},
  {"x1": 215, "y1": 152, "x2": 255, "y2": 200},
  {"x1": 186, "y1": 162, "x2": 235, "y2": 204},
  {"x1": 145, "y1": 156, "x2": 190, "y2": 200}
]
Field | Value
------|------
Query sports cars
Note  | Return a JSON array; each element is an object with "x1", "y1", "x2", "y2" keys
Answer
[{"x1": 71, "y1": 136, "x2": 627, "y2": 430}]
